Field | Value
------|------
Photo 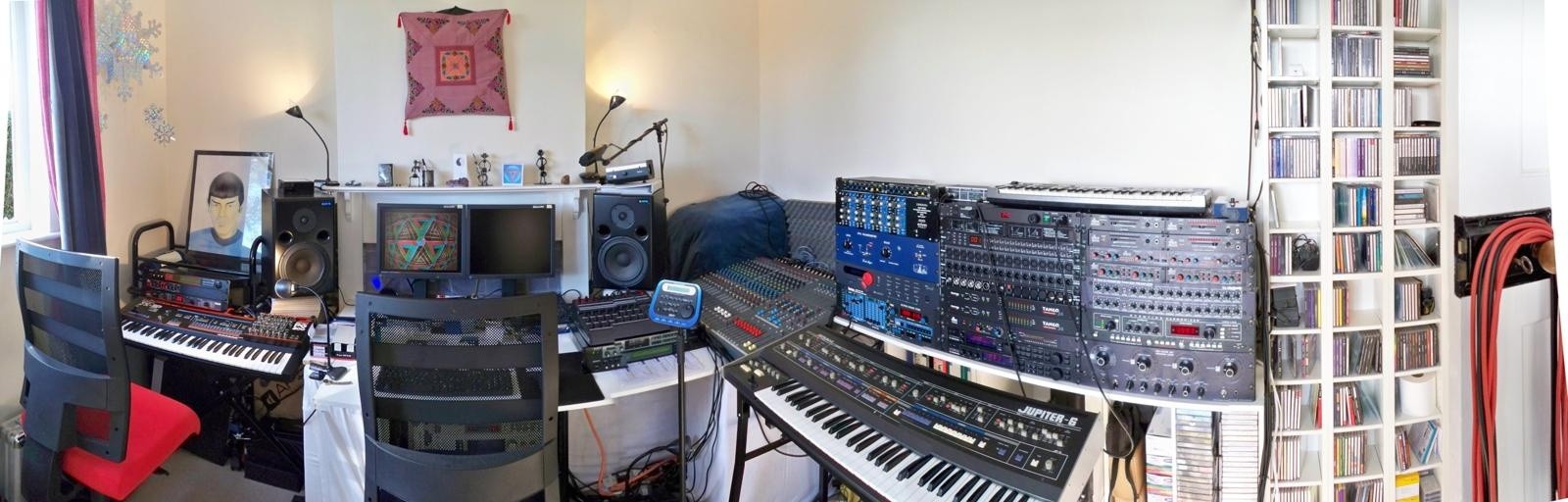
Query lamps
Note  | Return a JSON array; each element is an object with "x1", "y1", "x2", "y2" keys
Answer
[
  {"x1": 592, "y1": 95, "x2": 626, "y2": 185},
  {"x1": 286, "y1": 105, "x2": 340, "y2": 186}
]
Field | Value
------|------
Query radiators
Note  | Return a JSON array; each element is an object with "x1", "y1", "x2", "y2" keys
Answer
[{"x1": 0, "y1": 412, "x2": 37, "y2": 502}]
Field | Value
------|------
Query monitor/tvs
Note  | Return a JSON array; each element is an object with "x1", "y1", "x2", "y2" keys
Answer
[
  {"x1": 376, "y1": 206, "x2": 465, "y2": 297},
  {"x1": 468, "y1": 203, "x2": 555, "y2": 296}
]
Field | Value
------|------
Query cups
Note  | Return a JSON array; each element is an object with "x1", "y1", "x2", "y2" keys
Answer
[{"x1": 423, "y1": 170, "x2": 435, "y2": 187}]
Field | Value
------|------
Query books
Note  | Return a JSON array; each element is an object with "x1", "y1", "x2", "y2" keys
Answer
[{"x1": 1144, "y1": 0, "x2": 1443, "y2": 502}]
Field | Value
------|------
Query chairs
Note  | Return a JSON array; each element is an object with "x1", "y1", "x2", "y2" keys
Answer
[
  {"x1": 346, "y1": 293, "x2": 563, "y2": 502},
  {"x1": 16, "y1": 238, "x2": 203, "y2": 502}
]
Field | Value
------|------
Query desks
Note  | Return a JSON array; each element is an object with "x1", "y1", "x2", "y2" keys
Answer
[{"x1": 300, "y1": 184, "x2": 728, "y2": 502}]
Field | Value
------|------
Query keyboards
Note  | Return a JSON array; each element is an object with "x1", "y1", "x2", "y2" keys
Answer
[{"x1": 374, "y1": 368, "x2": 521, "y2": 400}]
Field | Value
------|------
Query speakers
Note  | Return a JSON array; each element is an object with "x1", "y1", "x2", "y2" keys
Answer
[
  {"x1": 588, "y1": 189, "x2": 666, "y2": 291},
  {"x1": 260, "y1": 189, "x2": 338, "y2": 295}
]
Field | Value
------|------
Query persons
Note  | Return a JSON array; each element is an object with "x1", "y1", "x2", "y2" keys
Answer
[{"x1": 188, "y1": 173, "x2": 261, "y2": 259}]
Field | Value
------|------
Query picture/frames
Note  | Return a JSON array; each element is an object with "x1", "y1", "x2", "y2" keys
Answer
[
  {"x1": 185, "y1": 150, "x2": 276, "y2": 263},
  {"x1": 502, "y1": 163, "x2": 524, "y2": 186}
]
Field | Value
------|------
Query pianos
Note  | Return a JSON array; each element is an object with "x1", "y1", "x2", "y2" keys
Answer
[
  {"x1": 727, "y1": 325, "x2": 1104, "y2": 501},
  {"x1": 986, "y1": 181, "x2": 1212, "y2": 220},
  {"x1": 119, "y1": 316, "x2": 292, "y2": 378}
]
